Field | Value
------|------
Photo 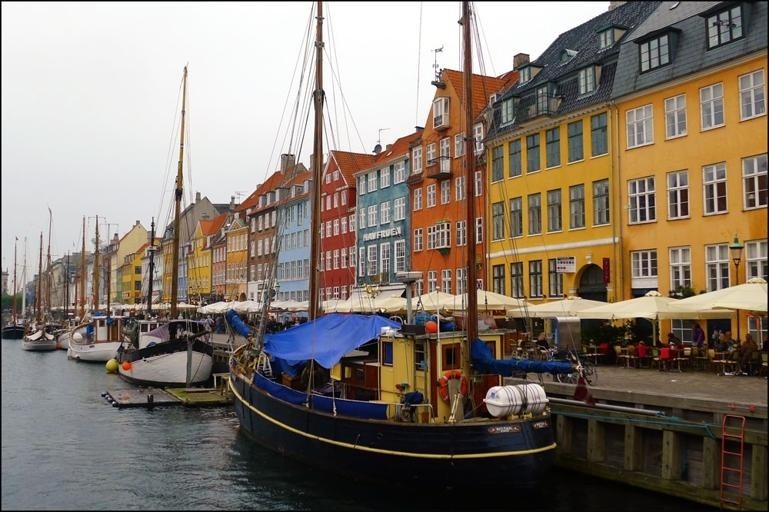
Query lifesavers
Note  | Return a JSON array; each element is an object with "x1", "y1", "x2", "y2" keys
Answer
[{"x1": 439, "y1": 370, "x2": 469, "y2": 406}]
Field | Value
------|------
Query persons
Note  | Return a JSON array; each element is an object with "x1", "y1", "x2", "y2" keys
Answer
[{"x1": 599, "y1": 323, "x2": 757, "y2": 376}]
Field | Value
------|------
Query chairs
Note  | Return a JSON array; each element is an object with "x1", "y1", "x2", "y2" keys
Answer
[{"x1": 577, "y1": 343, "x2": 769, "y2": 377}]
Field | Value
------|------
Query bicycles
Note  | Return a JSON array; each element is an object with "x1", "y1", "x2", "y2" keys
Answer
[{"x1": 548, "y1": 346, "x2": 599, "y2": 386}]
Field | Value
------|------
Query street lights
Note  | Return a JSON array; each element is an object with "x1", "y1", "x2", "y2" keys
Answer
[{"x1": 728, "y1": 232, "x2": 743, "y2": 346}]
